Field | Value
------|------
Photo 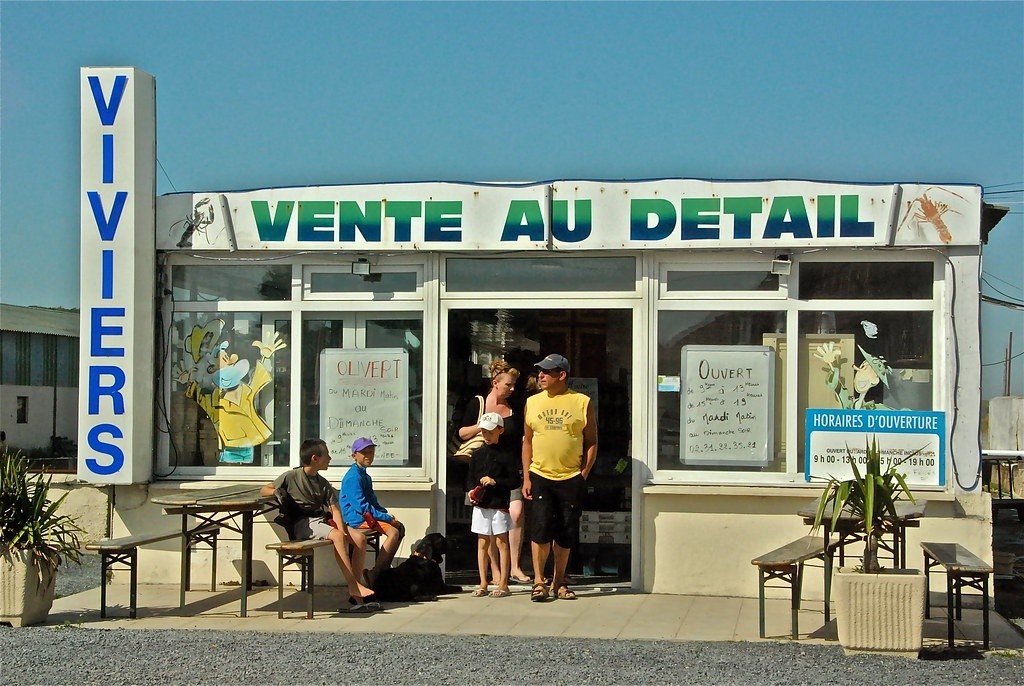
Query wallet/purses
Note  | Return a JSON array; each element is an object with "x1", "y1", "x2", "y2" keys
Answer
[{"x1": 469, "y1": 486, "x2": 484, "y2": 504}]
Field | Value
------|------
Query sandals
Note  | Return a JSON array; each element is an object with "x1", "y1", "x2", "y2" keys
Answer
[
  {"x1": 547, "y1": 581, "x2": 575, "y2": 599},
  {"x1": 531, "y1": 583, "x2": 549, "y2": 601}
]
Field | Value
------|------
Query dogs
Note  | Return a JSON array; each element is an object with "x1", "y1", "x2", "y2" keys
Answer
[{"x1": 374, "y1": 532, "x2": 464, "y2": 602}]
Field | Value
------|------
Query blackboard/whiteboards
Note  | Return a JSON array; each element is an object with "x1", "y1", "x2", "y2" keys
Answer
[
  {"x1": 320, "y1": 348, "x2": 409, "y2": 466},
  {"x1": 680, "y1": 344, "x2": 775, "y2": 466}
]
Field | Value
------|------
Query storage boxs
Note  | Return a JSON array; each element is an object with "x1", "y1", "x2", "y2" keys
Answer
[{"x1": 577, "y1": 509, "x2": 632, "y2": 546}]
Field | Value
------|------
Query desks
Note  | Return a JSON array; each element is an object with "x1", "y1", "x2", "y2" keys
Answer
[
  {"x1": 982, "y1": 448, "x2": 1024, "y2": 499},
  {"x1": 150, "y1": 482, "x2": 280, "y2": 618},
  {"x1": 797, "y1": 494, "x2": 928, "y2": 570}
]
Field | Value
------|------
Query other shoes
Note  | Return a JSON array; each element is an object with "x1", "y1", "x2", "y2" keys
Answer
[
  {"x1": 552, "y1": 574, "x2": 577, "y2": 585},
  {"x1": 363, "y1": 567, "x2": 376, "y2": 589}
]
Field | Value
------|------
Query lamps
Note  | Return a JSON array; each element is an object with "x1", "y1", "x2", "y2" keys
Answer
[
  {"x1": 353, "y1": 256, "x2": 370, "y2": 278},
  {"x1": 771, "y1": 253, "x2": 793, "y2": 276}
]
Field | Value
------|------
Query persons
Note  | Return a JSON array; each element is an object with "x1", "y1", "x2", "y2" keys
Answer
[
  {"x1": 259, "y1": 437, "x2": 374, "y2": 606},
  {"x1": 338, "y1": 438, "x2": 405, "y2": 590},
  {"x1": 467, "y1": 412, "x2": 521, "y2": 599},
  {"x1": 521, "y1": 353, "x2": 599, "y2": 602},
  {"x1": 450, "y1": 359, "x2": 532, "y2": 585}
]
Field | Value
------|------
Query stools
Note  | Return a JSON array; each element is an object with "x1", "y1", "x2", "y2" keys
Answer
[{"x1": 580, "y1": 543, "x2": 631, "y2": 583}]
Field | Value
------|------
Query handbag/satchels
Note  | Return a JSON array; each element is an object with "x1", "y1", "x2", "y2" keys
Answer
[{"x1": 447, "y1": 423, "x2": 485, "y2": 463}]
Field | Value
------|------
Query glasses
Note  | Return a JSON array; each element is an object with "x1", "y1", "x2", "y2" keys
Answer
[
  {"x1": 538, "y1": 365, "x2": 552, "y2": 374},
  {"x1": 491, "y1": 366, "x2": 517, "y2": 381}
]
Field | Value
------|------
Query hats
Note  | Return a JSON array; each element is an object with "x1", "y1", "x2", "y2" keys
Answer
[
  {"x1": 352, "y1": 438, "x2": 377, "y2": 454},
  {"x1": 477, "y1": 412, "x2": 504, "y2": 431},
  {"x1": 533, "y1": 354, "x2": 570, "y2": 374}
]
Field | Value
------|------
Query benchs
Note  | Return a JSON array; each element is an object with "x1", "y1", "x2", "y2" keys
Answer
[
  {"x1": 84, "y1": 521, "x2": 221, "y2": 618},
  {"x1": 920, "y1": 542, "x2": 993, "y2": 648},
  {"x1": 750, "y1": 535, "x2": 846, "y2": 640},
  {"x1": 265, "y1": 528, "x2": 388, "y2": 620}
]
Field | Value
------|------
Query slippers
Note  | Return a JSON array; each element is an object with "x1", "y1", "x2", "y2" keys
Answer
[
  {"x1": 488, "y1": 589, "x2": 512, "y2": 598},
  {"x1": 336, "y1": 602, "x2": 383, "y2": 613},
  {"x1": 470, "y1": 589, "x2": 489, "y2": 597},
  {"x1": 509, "y1": 574, "x2": 533, "y2": 583}
]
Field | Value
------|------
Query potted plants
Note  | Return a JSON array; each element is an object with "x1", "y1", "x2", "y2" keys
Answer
[
  {"x1": 0, "y1": 440, "x2": 91, "y2": 628},
  {"x1": 824, "y1": 433, "x2": 929, "y2": 660}
]
